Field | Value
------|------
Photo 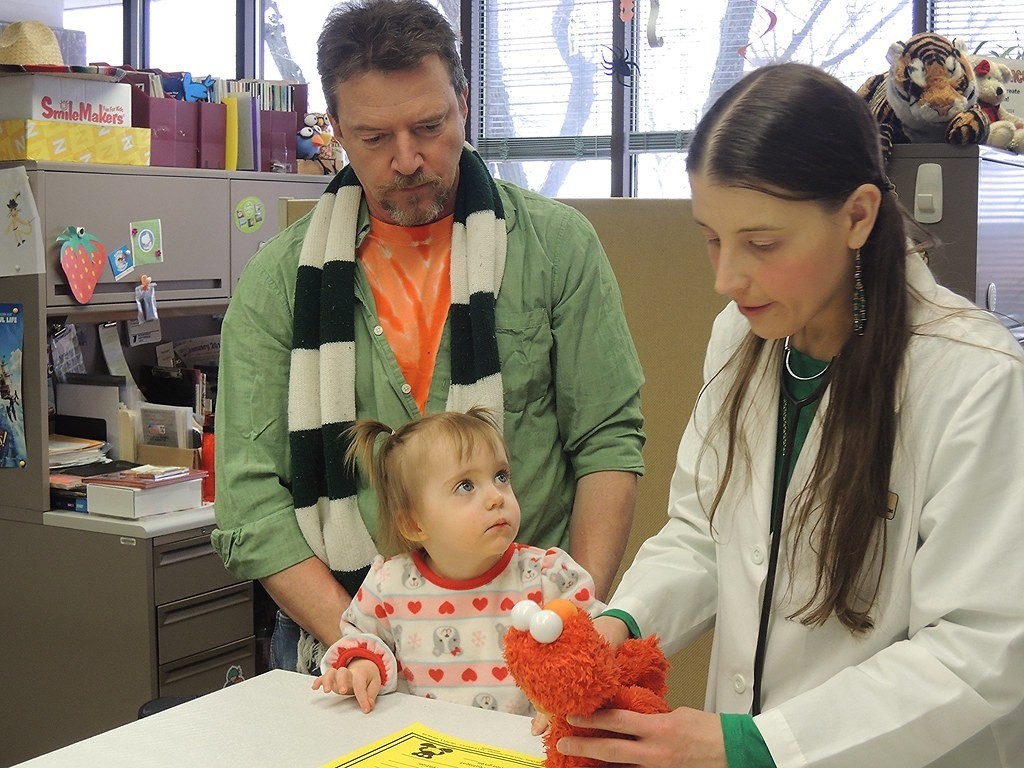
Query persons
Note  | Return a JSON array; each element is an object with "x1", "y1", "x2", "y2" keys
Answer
[
  {"x1": 311, "y1": 404, "x2": 608, "y2": 719},
  {"x1": 534, "y1": 62, "x2": 1024, "y2": 768},
  {"x1": 212, "y1": 0, "x2": 645, "y2": 678}
]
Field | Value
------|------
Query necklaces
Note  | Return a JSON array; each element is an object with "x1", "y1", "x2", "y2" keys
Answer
[{"x1": 785, "y1": 335, "x2": 838, "y2": 381}]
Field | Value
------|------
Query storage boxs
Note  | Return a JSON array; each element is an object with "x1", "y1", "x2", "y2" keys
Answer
[
  {"x1": 0, "y1": 22, "x2": 350, "y2": 176},
  {"x1": 86, "y1": 478, "x2": 202, "y2": 519},
  {"x1": 138, "y1": 444, "x2": 204, "y2": 470}
]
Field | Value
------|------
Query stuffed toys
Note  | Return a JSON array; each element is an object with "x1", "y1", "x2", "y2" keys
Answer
[
  {"x1": 303, "y1": 111, "x2": 331, "y2": 137},
  {"x1": 296, "y1": 127, "x2": 324, "y2": 160},
  {"x1": 856, "y1": 32, "x2": 989, "y2": 162},
  {"x1": 505, "y1": 599, "x2": 672, "y2": 768},
  {"x1": 968, "y1": 58, "x2": 1024, "y2": 154}
]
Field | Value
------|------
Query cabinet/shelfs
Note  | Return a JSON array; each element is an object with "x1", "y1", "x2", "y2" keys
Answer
[
  {"x1": 0, "y1": 158, "x2": 337, "y2": 768},
  {"x1": 886, "y1": 143, "x2": 1024, "y2": 345}
]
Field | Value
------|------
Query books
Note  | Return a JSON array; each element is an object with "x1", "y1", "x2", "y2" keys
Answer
[
  {"x1": 192, "y1": 76, "x2": 294, "y2": 112},
  {"x1": 80, "y1": 464, "x2": 209, "y2": 489},
  {"x1": 126, "y1": 70, "x2": 163, "y2": 98},
  {"x1": 148, "y1": 366, "x2": 206, "y2": 417}
]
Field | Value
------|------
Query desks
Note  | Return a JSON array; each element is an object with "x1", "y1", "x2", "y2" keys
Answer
[{"x1": 9, "y1": 669, "x2": 546, "y2": 768}]
